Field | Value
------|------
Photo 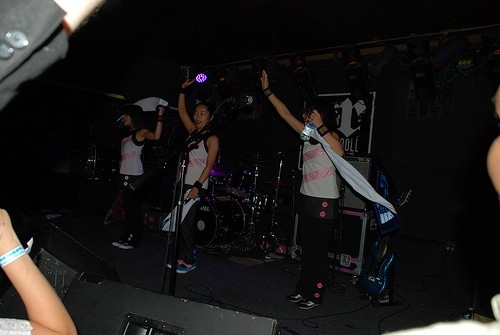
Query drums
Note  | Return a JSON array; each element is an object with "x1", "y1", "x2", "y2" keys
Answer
[
  {"x1": 208, "y1": 178, "x2": 245, "y2": 205},
  {"x1": 180, "y1": 195, "x2": 246, "y2": 251},
  {"x1": 244, "y1": 192, "x2": 277, "y2": 227}
]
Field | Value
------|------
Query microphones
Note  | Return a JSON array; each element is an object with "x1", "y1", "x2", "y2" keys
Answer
[{"x1": 227, "y1": 95, "x2": 253, "y2": 105}]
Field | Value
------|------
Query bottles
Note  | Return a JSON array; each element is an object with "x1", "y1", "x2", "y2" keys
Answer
[{"x1": 300, "y1": 121, "x2": 314, "y2": 141}]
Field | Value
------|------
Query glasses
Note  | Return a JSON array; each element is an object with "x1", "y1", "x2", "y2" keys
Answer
[{"x1": 302, "y1": 112, "x2": 308, "y2": 118}]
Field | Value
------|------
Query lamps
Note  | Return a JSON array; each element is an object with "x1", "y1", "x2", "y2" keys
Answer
[
  {"x1": 341, "y1": 43, "x2": 362, "y2": 66},
  {"x1": 428, "y1": 46, "x2": 461, "y2": 69},
  {"x1": 289, "y1": 51, "x2": 307, "y2": 74}
]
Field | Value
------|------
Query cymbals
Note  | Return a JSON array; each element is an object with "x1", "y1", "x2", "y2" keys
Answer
[
  {"x1": 273, "y1": 150, "x2": 300, "y2": 160},
  {"x1": 238, "y1": 157, "x2": 270, "y2": 165},
  {"x1": 264, "y1": 180, "x2": 297, "y2": 187}
]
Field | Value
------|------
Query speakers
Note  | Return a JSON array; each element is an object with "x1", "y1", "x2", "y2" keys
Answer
[
  {"x1": 1, "y1": 205, "x2": 120, "y2": 285},
  {"x1": 0, "y1": 248, "x2": 78, "y2": 320},
  {"x1": 335, "y1": 154, "x2": 371, "y2": 210},
  {"x1": 63, "y1": 272, "x2": 278, "y2": 334}
]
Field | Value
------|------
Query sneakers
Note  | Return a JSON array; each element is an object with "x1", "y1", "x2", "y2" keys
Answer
[
  {"x1": 286, "y1": 291, "x2": 304, "y2": 303},
  {"x1": 296, "y1": 298, "x2": 320, "y2": 310}
]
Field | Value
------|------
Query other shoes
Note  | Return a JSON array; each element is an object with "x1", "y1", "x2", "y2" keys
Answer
[
  {"x1": 112, "y1": 240, "x2": 135, "y2": 249},
  {"x1": 176, "y1": 262, "x2": 196, "y2": 274},
  {"x1": 166, "y1": 260, "x2": 184, "y2": 269}
]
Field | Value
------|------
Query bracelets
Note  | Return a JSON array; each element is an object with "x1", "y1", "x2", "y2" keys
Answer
[
  {"x1": 264, "y1": 87, "x2": 272, "y2": 97},
  {"x1": 179, "y1": 87, "x2": 186, "y2": 94},
  {"x1": 0, "y1": 237, "x2": 34, "y2": 267},
  {"x1": 157, "y1": 114, "x2": 163, "y2": 122},
  {"x1": 316, "y1": 124, "x2": 330, "y2": 137},
  {"x1": 193, "y1": 181, "x2": 203, "y2": 190}
]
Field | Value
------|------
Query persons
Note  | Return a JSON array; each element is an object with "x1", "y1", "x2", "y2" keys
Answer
[
  {"x1": 164, "y1": 78, "x2": 219, "y2": 273},
  {"x1": 110, "y1": 104, "x2": 166, "y2": 249},
  {"x1": 0, "y1": 209, "x2": 77, "y2": 335},
  {"x1": 0, "y1": 0, "x2": 102, "y2": 105},
  {"x1": 261, "y1": 69, "x2": 343, "y2": 309}
]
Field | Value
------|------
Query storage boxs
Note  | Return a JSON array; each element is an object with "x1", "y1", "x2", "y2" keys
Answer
[{"x1": 291, "y1": 210, "x2": 379, "y2": 284}]
